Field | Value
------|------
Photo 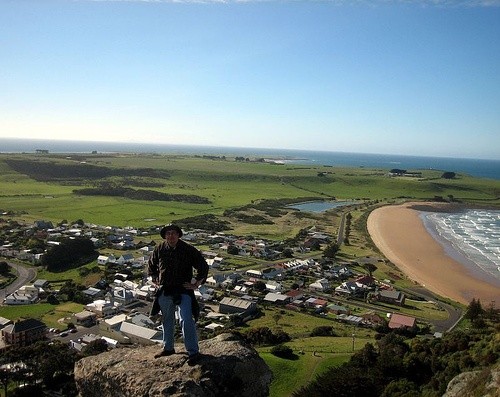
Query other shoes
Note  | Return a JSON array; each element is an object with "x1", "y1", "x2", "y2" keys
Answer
[
  {"x1": 154, "y1": 348, "x2": 175, "y2": 358},
  {"x1": 186, "y1": 351, "x2": 200, "y2": 366}
]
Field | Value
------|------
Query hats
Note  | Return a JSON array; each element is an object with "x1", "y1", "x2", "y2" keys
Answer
[{"x1": 160, "y1": 224, "x2": 183, "y2": 239}]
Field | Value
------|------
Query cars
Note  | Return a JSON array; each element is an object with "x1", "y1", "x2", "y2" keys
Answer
[{"x1": 48, "y1": 326, "x2": 78, "y2": 337}]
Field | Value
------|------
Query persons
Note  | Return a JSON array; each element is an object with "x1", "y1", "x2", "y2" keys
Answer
[{"x1": 147, "y1": 223, "x2": 209, "y2": 363}]
